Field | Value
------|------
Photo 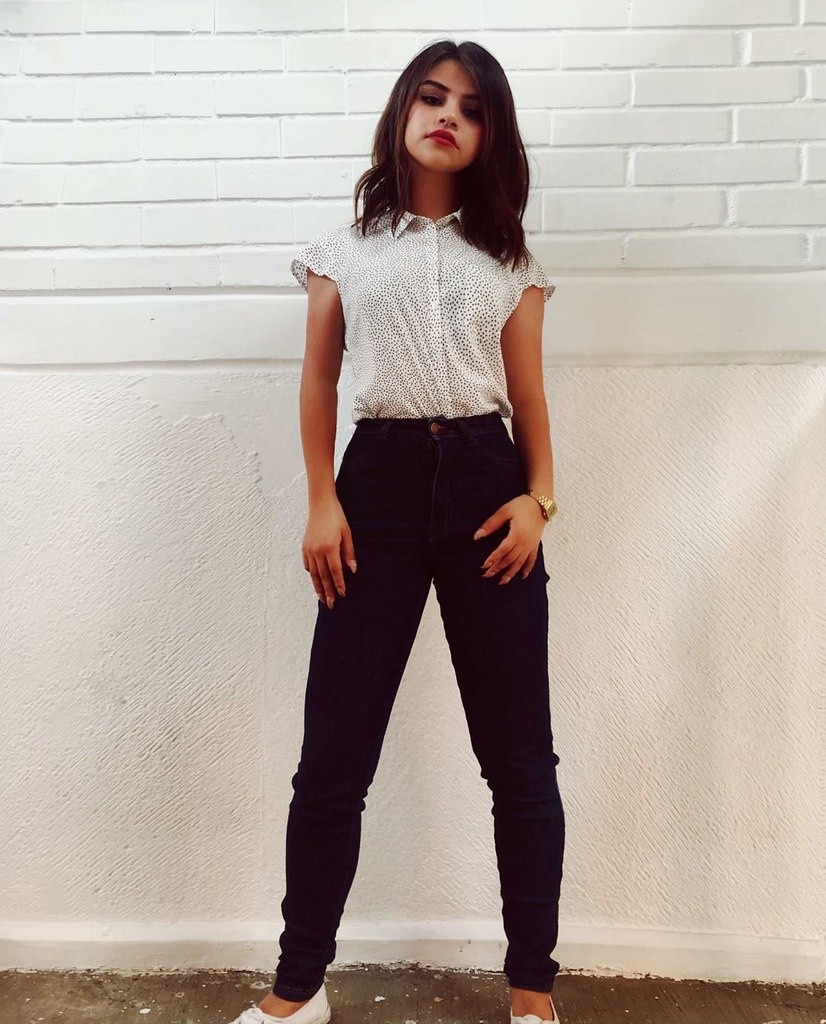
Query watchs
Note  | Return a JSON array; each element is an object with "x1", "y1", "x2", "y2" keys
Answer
[{"x1": 525, "y1": 488, "x2": 558, "y2": 522}]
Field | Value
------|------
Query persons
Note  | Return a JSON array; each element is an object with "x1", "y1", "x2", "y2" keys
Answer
[{"x1": 226, "y1": 40, "x2": 564, "y2": 1024}]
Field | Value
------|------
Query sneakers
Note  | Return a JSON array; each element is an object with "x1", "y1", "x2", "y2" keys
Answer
[
  {"x1": 510, "y1": 994, "x2": 559, "y2": 1024},
  {"x1": 228, "y1": 982, "x2": 331, "y2": 1024}
]
